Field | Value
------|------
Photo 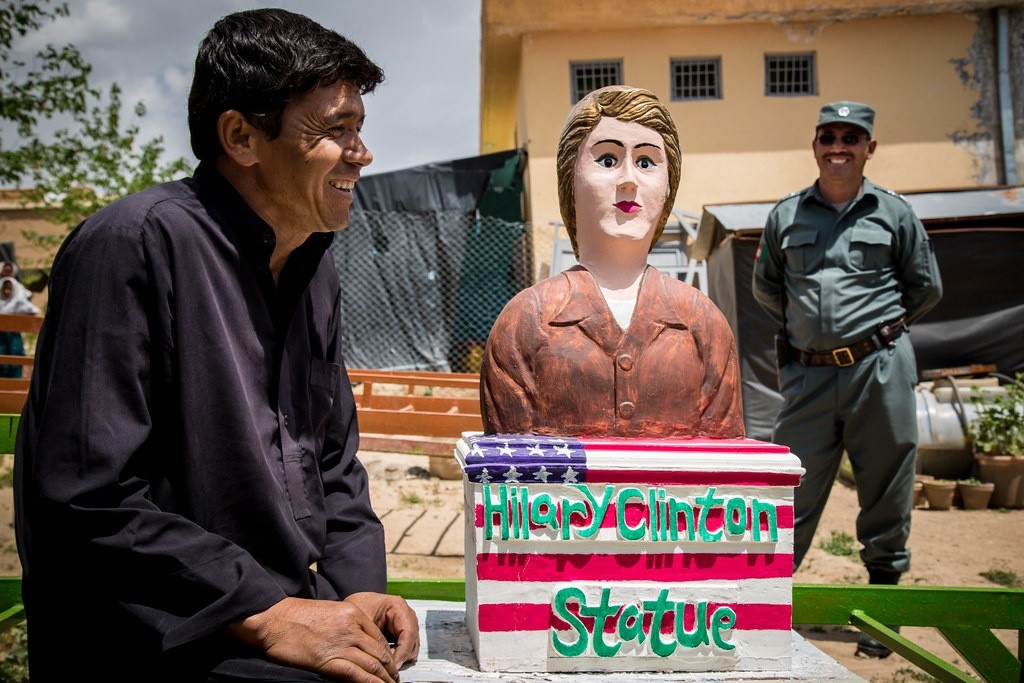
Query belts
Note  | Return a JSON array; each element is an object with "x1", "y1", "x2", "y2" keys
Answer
[{"x1": 779, "y1": 315, "x2": 909, "y2": 367}]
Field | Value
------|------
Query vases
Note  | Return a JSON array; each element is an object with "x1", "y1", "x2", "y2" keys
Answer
[
  {"x1": 913, "y1": 483, "x2": 924, "y2": 509},
  {"x1": 925, "y1": 478, "x2": 957, "y2": 509}
]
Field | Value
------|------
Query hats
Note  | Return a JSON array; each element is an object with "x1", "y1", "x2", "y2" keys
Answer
[{"x1": 816, "y1": 101, "x2": 876, "y2": 139}]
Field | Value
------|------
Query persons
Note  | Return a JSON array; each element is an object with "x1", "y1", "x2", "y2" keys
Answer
[
  {"x1": 480, "y1": 85, "x2": 746, "y2": 437},
  {"x1": 0, "y1": 262, "x2": 39, "y2": 378},
  {"x1": 13, "y1": 8, "x2": 421, "y2": 683},
  {"x1": 752, "y1": 100, "x2": 943, "y2": 657}
]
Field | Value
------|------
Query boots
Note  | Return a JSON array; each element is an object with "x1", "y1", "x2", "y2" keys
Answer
[{"x1": 856, "y1": 570, "x2": 900, "y2": 658}]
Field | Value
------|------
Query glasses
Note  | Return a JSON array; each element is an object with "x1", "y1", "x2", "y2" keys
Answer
[{"x1": 815, "y1": 131, "x2": 866, "y2": 145}]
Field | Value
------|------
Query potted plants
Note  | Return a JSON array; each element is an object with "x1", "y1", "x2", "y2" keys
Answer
[
  {"x1": 963, "y1": 371, "x2": 1024, "y2": 509},
  {"x1": 959, "y1": 477, "x2": 995, "y2": 509}
]
